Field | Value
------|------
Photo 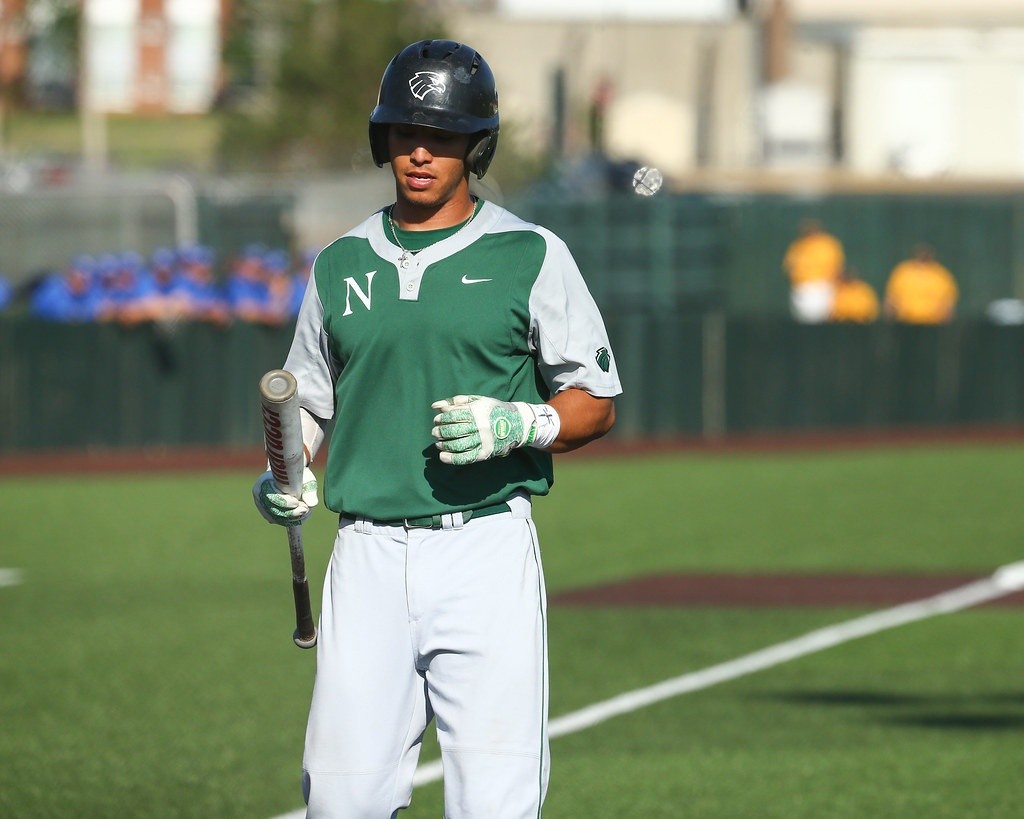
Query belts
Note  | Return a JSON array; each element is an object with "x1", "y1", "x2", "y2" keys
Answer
[{"x1": 337, "y1": 501, "x2": 510, "y2": 529}]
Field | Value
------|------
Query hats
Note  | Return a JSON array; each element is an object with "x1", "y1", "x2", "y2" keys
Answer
[{"x1": 70, "y1": 244, "x2": 318, "y2": 276}]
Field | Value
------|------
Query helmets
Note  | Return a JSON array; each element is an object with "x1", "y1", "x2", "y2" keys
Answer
[{"x1": 369, "y1": 41, "x2": 498, "y2": 179}]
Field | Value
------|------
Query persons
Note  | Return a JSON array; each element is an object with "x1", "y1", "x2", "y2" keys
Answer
[
  {"x1": 784, "y1": 218, "x2": 955, "y2": 324},
  {"x1": 252, "y1": 39, "x2": 624, "y2": 819},
  {"x1": 32, "y1": 244, "x2": 318, "y2": 326}
]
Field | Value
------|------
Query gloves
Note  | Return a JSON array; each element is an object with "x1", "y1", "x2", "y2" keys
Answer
[
  {"x1": 252, "y1": 466, "x2": 318, "y2": 526},
  {"x1": 430, "y1": 395, "x2": 536, "y2": 465}
]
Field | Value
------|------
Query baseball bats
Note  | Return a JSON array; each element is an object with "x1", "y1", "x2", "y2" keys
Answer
[{"x1": 257, "y1": 369, "x2": 317, "y2": 648}]
